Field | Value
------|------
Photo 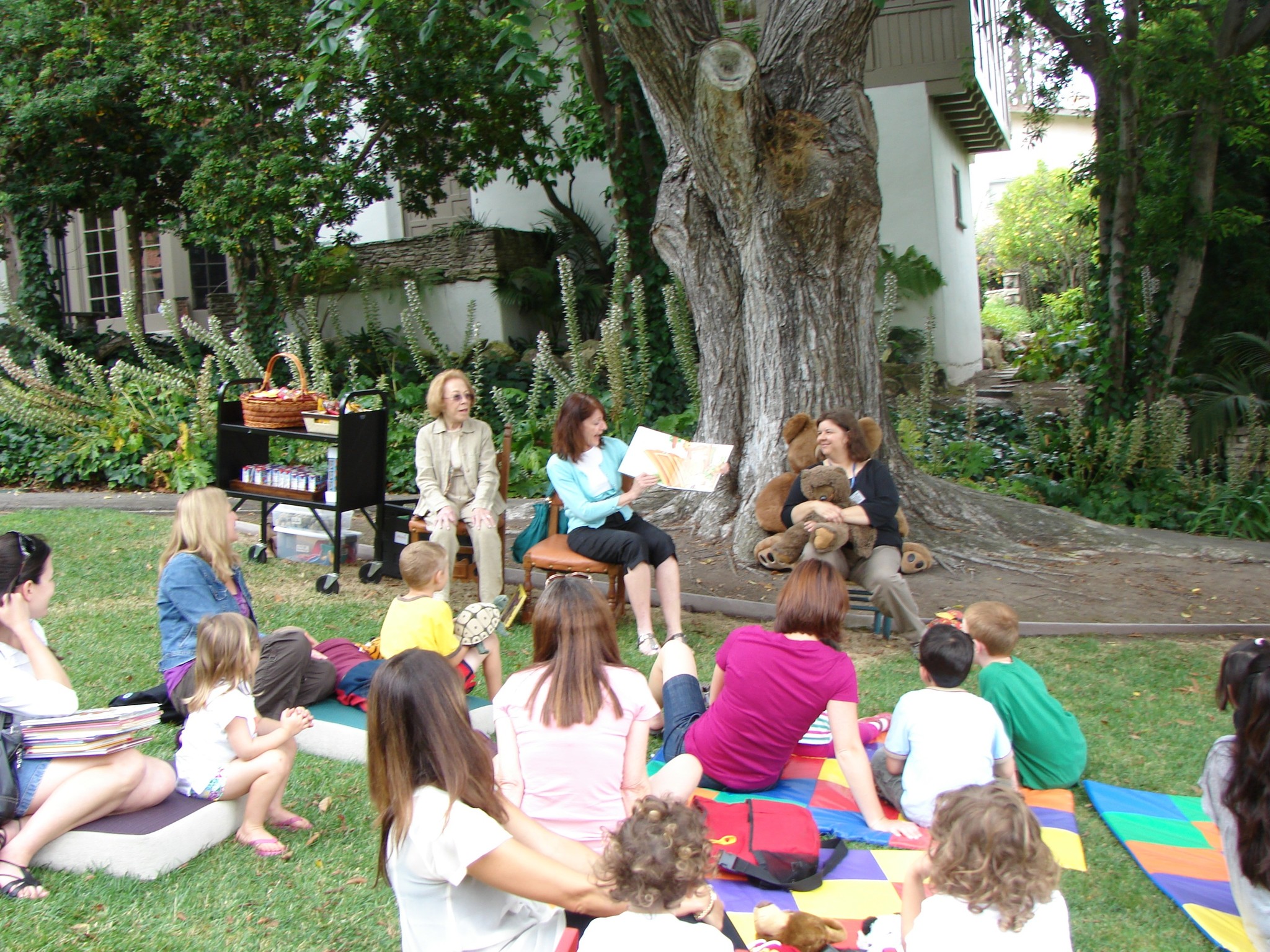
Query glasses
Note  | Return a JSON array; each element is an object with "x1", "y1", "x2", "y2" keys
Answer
[
  {"x1": 5, "y1": 530, "x2": 36, "y2": 593},
  {"x1": 442, "y1": 393, "x2": 476, "y2": 401}
]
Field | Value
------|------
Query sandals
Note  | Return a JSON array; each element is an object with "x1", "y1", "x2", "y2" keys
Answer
[
  {"x1": 857, "y1": 714, "x2": 893, "y2": 732},
  {"x1": 1, "y1": 859, "x2": 45, "y2": 901},
  {"x1": 665, "y1": 633, "x2": 696, "y2": 655},
  {"x1": 636, "y1": 633, "x2": 661, "y2": 655}
]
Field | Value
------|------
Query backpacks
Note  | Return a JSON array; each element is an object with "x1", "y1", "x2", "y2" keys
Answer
[{"x1": 689, "y1": 799, "x2": 850, "y2": 889}]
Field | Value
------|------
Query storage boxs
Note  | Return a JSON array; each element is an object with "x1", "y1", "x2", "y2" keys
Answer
[
  {"x1": 271, "y1": 505, "x2": 354, "y2": 535},
  {"x1": 302, "y1": 409, "x2": 376, "y2": 436},
  {"x1": 273, "y1": 526, "x2": 362, "y2": 567}
]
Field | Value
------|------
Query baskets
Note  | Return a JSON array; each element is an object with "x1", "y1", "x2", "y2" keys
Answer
[{"x1": 240, "y1": 352, "x2": 324, "y2": 430}]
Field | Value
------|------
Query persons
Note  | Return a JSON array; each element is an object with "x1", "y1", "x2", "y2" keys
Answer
[
  {"x1": 412, "y1": 367, "x2": 505, "y2": 607},
  {"x1": 175, "y1": 610, "x2": 315, "y2": 855},
  {"x1": 376, "y1": 538, "x2": 505, "y2": 702},
  {"x1": 574, "y1": 792, "x2": 736, "y2": 952},
  {"x1": 869, "y1": 624, "x2": 1023, "y2": 830},
  {"x1": 647, "y1": 559, "x2": 926, "y2": 842},
  {"x1": 900, "y1": 779, "x2": 1074, "y2": 952},
  {"x1": 0, "y1": 528, "x2": 178, "y2": 902},
  {"x1": 780, "y1": 407, "x2": 928, "y2": 644},
  {"x1": 962, "y1": 599, "x2": 1089, "y2": 795},
  {"x1": 545, "y1": 390, "x2": 732, "y2": 657},
  {"x1": 489, "y1": 570, "x2": 706, "y2": 860},
  {"x1": 360, "y1": 645, "x2": 728, "y2": 952},
  {"x1": 154, "y1": 485, "x2": 338, "y2": 719},
  {"x1": 1198, "y1": 634, "x2": 1270, "y2": 952}
]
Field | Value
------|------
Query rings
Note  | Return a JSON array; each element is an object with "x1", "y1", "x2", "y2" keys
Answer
[{"x1": 486, "y1": 514, "x2": 491, "y2": 518}]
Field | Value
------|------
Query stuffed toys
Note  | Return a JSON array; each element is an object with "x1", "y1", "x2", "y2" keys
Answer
[
  {"x1": 770, "y1": 464, "x2": 877, "y2": 564},
  {"x1": 753, "y1": 411, "x2": 935, "y2": 576},
  {"x1": 748, "y1": 900, "x2": 848, "y2": 952},
  {"x1": 454, "y1": 593, "x2": 509, "y2": 655}
]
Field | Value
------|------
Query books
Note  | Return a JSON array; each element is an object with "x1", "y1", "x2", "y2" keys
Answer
[
  {"x1": 500, "y1": 582, "x2": 527, "y2": 629},
  {"x1": 615, "y1": 424, "x2": 736, "y2": 493},
  {"x1": 20, "y1": 701, "x2": 165, "y2": 761}
]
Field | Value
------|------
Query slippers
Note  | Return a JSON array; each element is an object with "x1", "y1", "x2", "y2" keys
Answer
[
  {"x1": 236, "y1": 834, "x2": 286, "y2": 855},
  {"x1": 273, "y1": 815, "x2": 315, "y2": 830}
]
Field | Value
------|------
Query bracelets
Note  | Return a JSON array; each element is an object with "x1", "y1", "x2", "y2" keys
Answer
[{"x1": 693, "y1": 884, "x2": 716, "y2": 921}]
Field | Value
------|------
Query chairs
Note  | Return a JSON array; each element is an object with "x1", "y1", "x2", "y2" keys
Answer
[
  {"x1": 522, "y1": 473, "x2": 633, "y2": 634},
  {"x1": 842, "y1": 579, "x2": 892, "y2": 642},
  {"x1": 410, "y1": 424, "x2": 511, "y2": 583}
]
